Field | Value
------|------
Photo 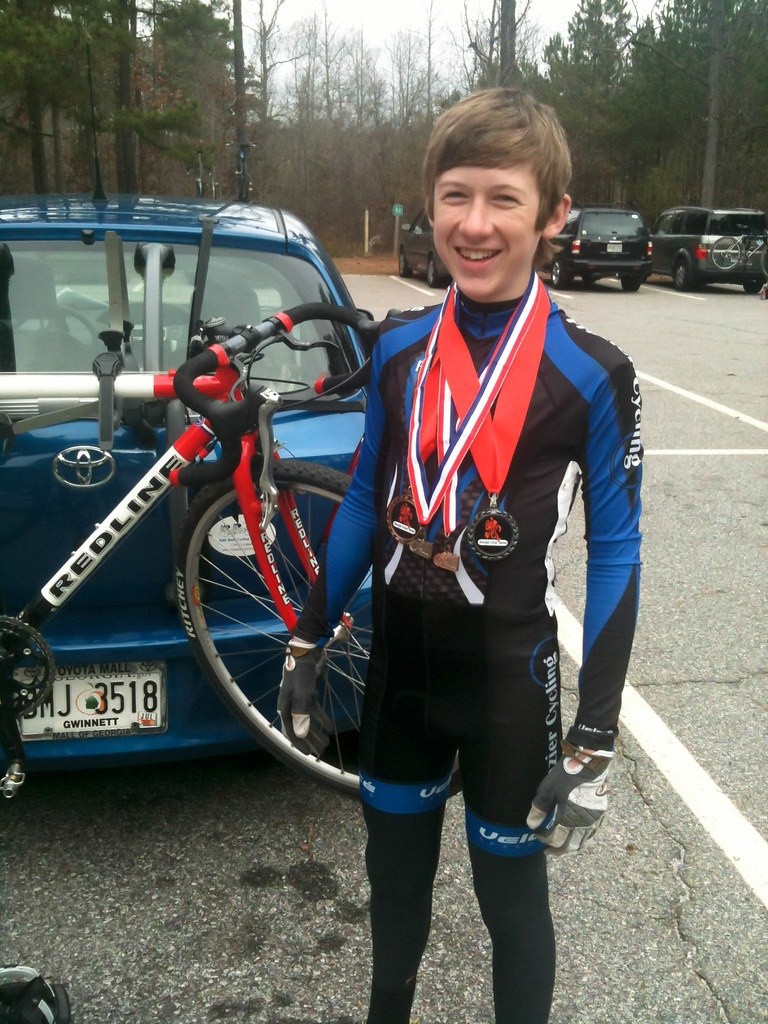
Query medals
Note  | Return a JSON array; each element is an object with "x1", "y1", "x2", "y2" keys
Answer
[{"x1": 385, "y1": 485, "x2": 521, "y2": 572}]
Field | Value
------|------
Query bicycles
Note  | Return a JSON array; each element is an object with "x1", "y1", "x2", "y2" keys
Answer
[
  {"x1": 710, "y1": 223, "x2": 767, "y2": 274},
  {"x1": 0, "y1": 306, "x2": 461, "y2": 798}
]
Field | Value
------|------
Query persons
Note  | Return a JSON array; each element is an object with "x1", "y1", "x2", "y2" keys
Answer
[{"x1": 275, "y1": 89, "x2": 642, "y2": 1023}]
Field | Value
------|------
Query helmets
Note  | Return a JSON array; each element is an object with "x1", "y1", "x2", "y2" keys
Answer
[{"x1": 0, "y1": 964, "x2": 70, "y2": 1024}]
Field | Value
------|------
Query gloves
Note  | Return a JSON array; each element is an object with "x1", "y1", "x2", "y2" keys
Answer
[
  {"x1": 526, "y1": 739, "x2": 615, "y2": 856},
  {"x1": 277, "y1": 639, "x2": 333, "y2": 755}
]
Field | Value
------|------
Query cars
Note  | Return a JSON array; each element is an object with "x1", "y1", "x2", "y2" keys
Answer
[
  {"x1": 398, "y1": 207, "x2": 454, "y2": 288},
  {"x1": 1, "y1": 193, "x2": 387, "y2": 798}
]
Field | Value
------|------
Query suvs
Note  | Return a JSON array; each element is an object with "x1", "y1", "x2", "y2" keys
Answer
[
  {"x1": 538, "y1": 203, "x2": 654, "y2": 291},
  {"x1": 647, "y1": 206, "x2": 768, "y2": 291}
]
